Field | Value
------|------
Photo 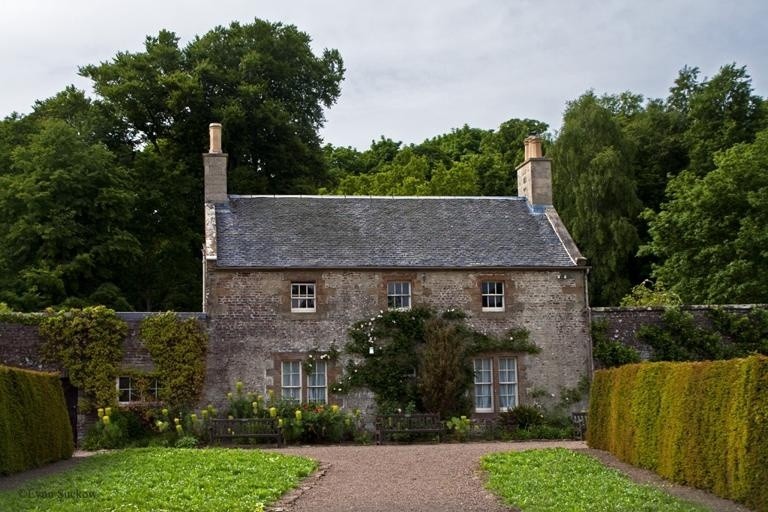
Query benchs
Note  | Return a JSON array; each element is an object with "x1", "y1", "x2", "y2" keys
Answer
[
  {"x1": 374, "y1": 413, "x2": 443, "y2": 446},
  {"x1": 571, "y1": 413, "x2": 587, "y2": 439},
  {"x1": 208, "y1": 417, "x2": 281, "y2": 448}
]
